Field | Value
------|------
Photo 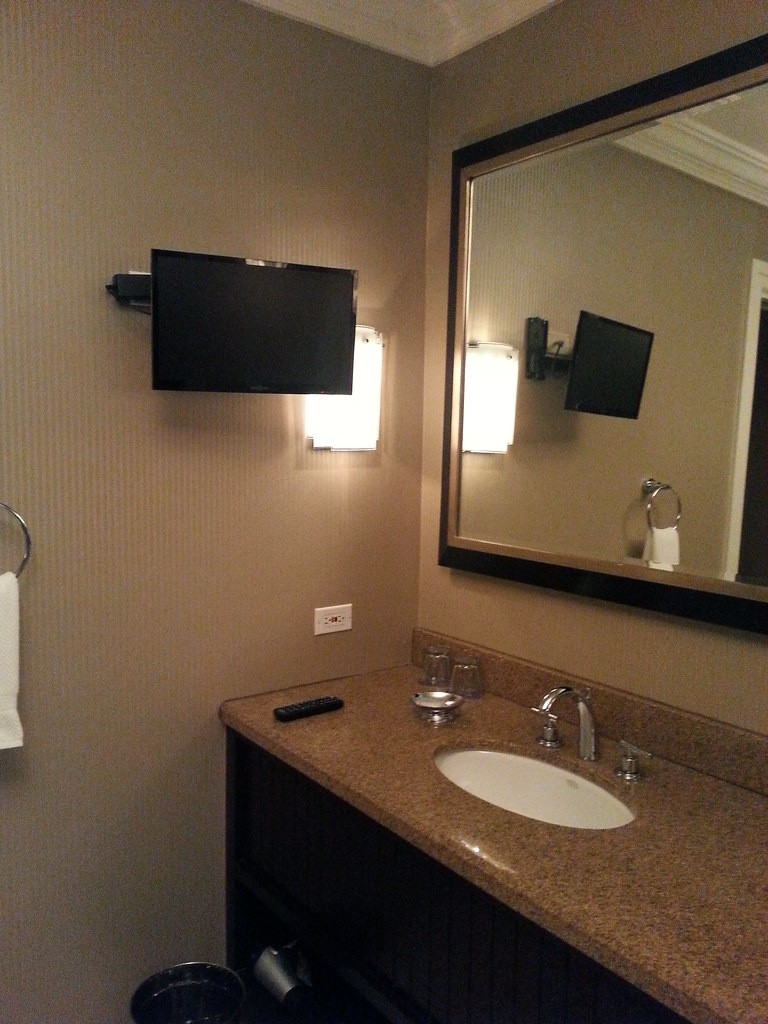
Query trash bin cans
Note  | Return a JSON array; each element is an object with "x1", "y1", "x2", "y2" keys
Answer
[{"x1": 127, "y1": 960, "x2": 248, "y2": 1024}]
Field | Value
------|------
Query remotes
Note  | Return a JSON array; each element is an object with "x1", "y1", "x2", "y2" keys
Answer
[{"x1": 272, "y1": 696, "x2": 344, "y2": 722}]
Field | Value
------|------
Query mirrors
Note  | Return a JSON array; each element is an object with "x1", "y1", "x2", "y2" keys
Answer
[{"x1": 438, "y1": 32, "x2": 767, "y2": 634}]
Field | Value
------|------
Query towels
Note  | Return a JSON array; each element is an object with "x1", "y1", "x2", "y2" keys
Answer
[
  {"x1": 640, "y1": 526, "x2": 681, "y2": 573},
  {"x1": 0, "y1": 571, "x2": 26, "y2": 750}
]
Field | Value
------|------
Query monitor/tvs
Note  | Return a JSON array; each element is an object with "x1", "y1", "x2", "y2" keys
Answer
[
  {"x1": 564, "y1": 309, "x2": 655, "y2": 420},
  {"x1": 148, "y1": 248, "x2": 358, "y2": 395}
]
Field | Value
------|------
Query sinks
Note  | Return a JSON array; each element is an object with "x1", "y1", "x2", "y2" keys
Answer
[{"x1": 430, "y1": 737, "x2": 640, "y2": 832}]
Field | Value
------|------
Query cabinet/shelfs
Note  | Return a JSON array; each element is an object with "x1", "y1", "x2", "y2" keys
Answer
[{"x1": 224, "y1": 727, "x2": 690, "y2": 1024}]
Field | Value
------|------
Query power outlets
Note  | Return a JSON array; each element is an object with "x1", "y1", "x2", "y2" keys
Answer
[{"x1": 314, "y1": 604, "x2": 352, "y2": 636}]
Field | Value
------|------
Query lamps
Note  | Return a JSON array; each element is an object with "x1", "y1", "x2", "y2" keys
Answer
[
  {"x1": 461, "y1": 342, "x2": 519, "y2": 454},
  {"x1": 304, "y1": 324, "x2": 383, "y2": 452}
]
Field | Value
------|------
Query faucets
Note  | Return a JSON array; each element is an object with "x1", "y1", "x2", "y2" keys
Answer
[{"x1": 529, "y1": 685, "x2": 604, "y2": 763}]
